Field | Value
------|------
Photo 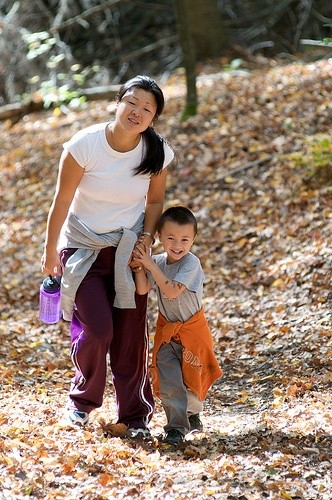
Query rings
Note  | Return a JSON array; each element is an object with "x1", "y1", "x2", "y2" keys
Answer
[{"x1": 139, "y1": 266, "x2": 143, "y2": 270}]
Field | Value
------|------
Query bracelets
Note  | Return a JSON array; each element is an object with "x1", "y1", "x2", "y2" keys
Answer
[{"x1": 142, "y1": 232, "x2": 155, "y2": 245}]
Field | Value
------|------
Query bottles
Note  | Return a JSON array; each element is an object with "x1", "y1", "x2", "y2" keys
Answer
[{"x1": 38, "y1": 275, "x2": 61, "y2": 324}]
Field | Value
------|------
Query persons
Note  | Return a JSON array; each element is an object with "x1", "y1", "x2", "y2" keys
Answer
[
  {"x1": 133, "y1": 207, "x2": 214, "y2": 446},
  {"x1": 41, "y1": 75, "x2": 175, "y2": 444}
]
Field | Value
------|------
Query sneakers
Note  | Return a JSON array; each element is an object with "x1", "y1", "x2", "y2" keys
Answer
[
  {"x1": 130, "y1": 427, "x2": 151, "y2": 440},
  {"x1": 187, "y1": 413, "x2": 203, "y2": 432},
  {"x1": 162, "y1": 429, "x2": 185, "y2": 449},
  {"x1": 68, "y1": 409, "x2": 90, "y2": 427}
]
